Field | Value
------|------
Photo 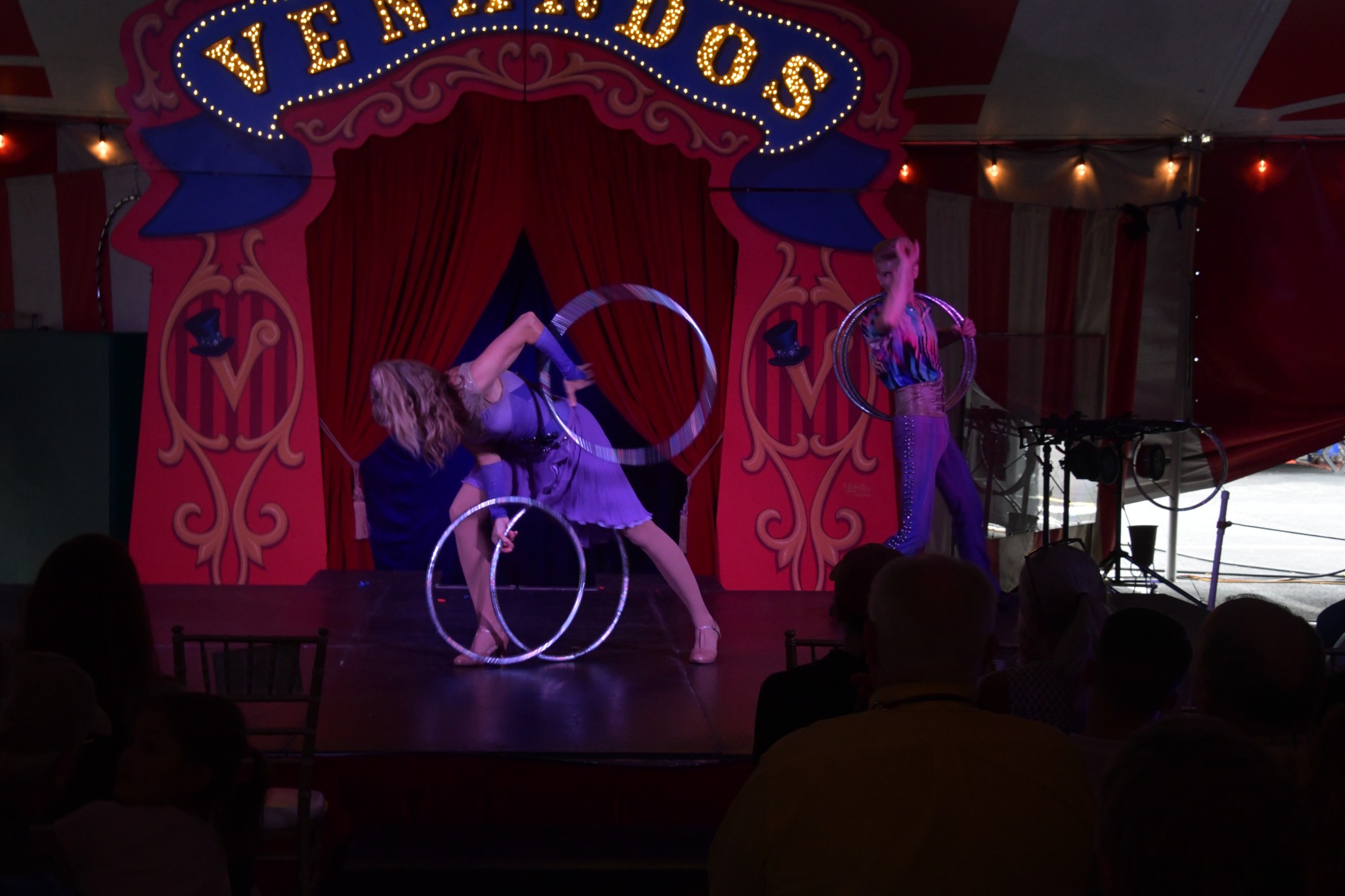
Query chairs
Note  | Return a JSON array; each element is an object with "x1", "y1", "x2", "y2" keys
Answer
[{"x1": 172, "y1": 625, "x2": 329, "y2": 836}]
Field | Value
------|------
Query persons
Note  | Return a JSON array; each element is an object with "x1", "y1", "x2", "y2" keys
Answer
[
  {"x1": 0, "y1": 532, "x2": 271, "y2": 895},
  {"x1": 698, "y1": 539, "x2": 1345, "y2": 896},
  {"x1": 857, "y1": 234, "x2": 1008, "y2": 596},
  {"x1": 368, "y1": 311, "x2": 718, "y2": 663}
]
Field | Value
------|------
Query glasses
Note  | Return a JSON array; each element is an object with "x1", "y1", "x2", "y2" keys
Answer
[{"x1": 1025, "y1": 537, "x2": 1091, "y2": 605}]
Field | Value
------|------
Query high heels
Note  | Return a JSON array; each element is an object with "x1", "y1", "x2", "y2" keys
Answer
[
  {"x1": 453, "y1": 628, "x2": 509, "y2": 667},
  {"x1": 689, "y1": 618, "x2": 722, "y2": 666}
]
